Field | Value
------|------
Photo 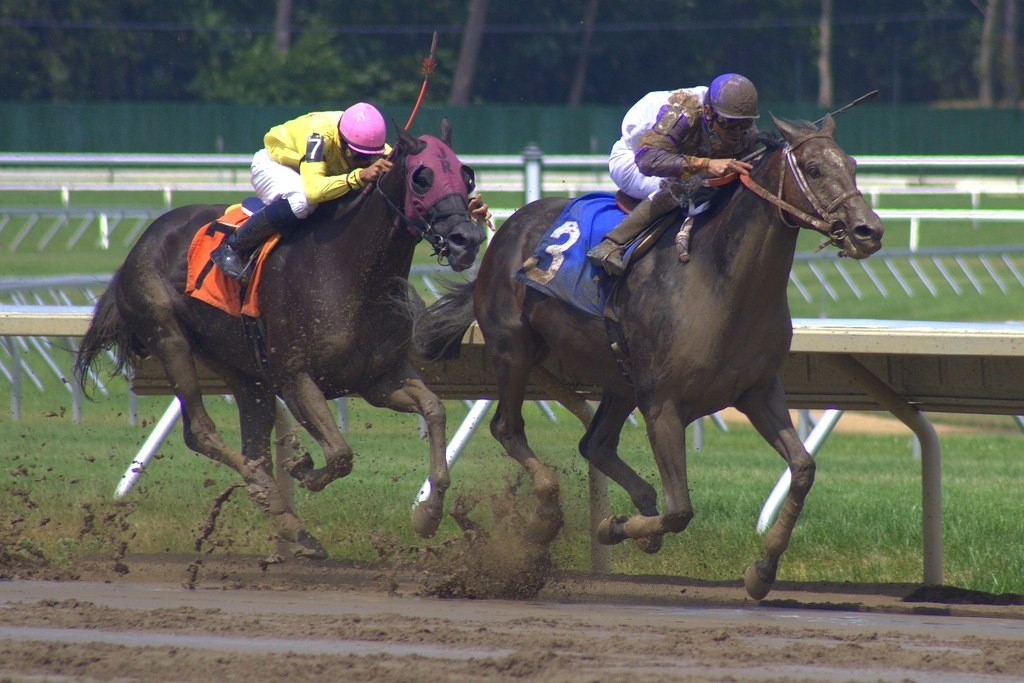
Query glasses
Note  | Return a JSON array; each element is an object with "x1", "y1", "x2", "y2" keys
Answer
[
  {"x1": 715, "y1": 115, "x2": 753, "y2": 129},
  {"x1": 345, "y1": 149, "x2": 382, "y2": 161}
]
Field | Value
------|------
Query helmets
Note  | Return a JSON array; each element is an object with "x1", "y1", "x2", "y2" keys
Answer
[
  {"x1": 704, "y1": 73, "x2": 761, "y2": 120},
  {"x1": 338, "y1": 102, "x2": 386, "y2": 154}
]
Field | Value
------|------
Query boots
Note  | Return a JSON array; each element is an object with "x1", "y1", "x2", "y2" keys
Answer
[
  {"x1": 207, "y1": 207, "x2": 277, "y2": 283},
  {"x1": 585, "y1": 193, "x2": 661, "y2": 275}
]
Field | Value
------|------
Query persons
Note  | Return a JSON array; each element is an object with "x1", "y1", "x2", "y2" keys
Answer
[
  {"x1": 585, "y1": 72, "x2": 772, "y2": 278},
  {"x1": 207, "y1": 100, "x2": 502, "y2": 295}
]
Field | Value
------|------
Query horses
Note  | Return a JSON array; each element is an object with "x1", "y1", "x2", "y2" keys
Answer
[
  {"x1": 71, "y1": 115, "x2": 489, "y2": 559},
  {"x1": 376, "y1": 108, "x2": 887, "y2": 601}
]
